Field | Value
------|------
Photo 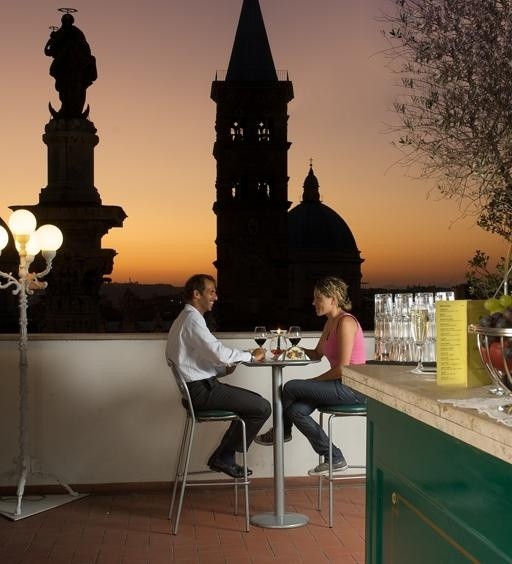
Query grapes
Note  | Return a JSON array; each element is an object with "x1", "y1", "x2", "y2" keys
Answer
[
  {"x1": 477, "y1": 293, "x2": 512, "y2": 358},
  {"x1": 504, "y1": 373, "x2": 512, "y2": 391}
]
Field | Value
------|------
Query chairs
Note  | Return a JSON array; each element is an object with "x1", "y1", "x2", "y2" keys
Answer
[{"x1": 165, "y1": 357, "x2": 251, "y2": 536}]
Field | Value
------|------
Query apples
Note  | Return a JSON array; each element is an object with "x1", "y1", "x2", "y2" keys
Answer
[{"x1": 480, "y1": 341, "x2": 512, "y2": 374}]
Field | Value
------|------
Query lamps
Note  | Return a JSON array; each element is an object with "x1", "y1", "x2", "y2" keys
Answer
[{"x1": 0, "y1": 210, "x2": 89, "y2": 523}]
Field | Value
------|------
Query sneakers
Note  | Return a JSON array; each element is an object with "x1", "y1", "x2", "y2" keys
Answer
[
  {"x1": 308, "y1": 461, "x2": 348, "y2": 476},
  {"x1": 253, "y1": 427, "x2": 292, "y2": 446}
]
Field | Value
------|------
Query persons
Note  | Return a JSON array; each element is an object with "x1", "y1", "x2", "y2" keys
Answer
[
  {"x1": 165, "y1": 274, "x2": 271, "y2": 478},
  {"x1": 254, "y1": 276, "x2": 366, "y2": 476}
]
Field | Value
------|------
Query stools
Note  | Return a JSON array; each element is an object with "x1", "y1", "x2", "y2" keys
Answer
[{"x1": 321, "y1": 400, "x2": 366, "y2": 528}]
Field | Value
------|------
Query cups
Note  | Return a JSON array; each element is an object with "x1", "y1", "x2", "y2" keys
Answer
[{"x1": 374, "y1": 292, "x2": 455, "y2": 362}]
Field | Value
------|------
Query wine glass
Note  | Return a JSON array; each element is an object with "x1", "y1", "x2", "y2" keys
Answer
[
  {"x1": 409, "y1": 303, "x2": 430, "y2": 375},
  {"x1": 287, "y1": 326, "x2": 302, "y2": 347},
  {"x1": 253, "y1": 326, "x2": 268, "y2": 348}
]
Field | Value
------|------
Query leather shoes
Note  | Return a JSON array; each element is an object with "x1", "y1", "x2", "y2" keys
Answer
[{"x1": 206, "y1": 449, "x2": 252, "y2": 478}]
